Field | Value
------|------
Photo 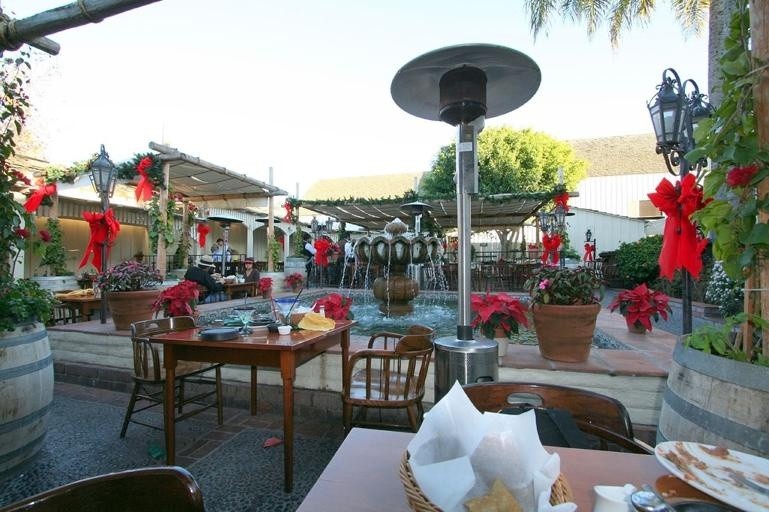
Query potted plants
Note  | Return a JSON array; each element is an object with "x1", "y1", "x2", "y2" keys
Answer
[
  {"x1": 283, "y1": 272, "x2": 305, "y2": 293},
  {"x1": 285, "y1": 224, "x2": 308, "y2": 278},
  {"x1": 95, "y1": 260, "x2": 165, "y2": 330},
  {"x1": 606, "y1": 284, "x2": 673, "y2": 333},
  {"x1": 470, "y1": 288, "x2": 530, "y2": 357},
  {"x1": 259, "y1": 278, "x2": 273, "y2": 299}
]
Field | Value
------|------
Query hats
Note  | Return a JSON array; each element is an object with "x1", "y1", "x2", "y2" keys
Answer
[
  {"x1": 195, "y1": 255, "x2": 216, "y2": 268},
  {"x1": 243, "y1": 257, "x2": 254, "y2": 263}
]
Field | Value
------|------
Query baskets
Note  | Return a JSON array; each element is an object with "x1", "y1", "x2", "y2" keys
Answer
[
  {"x1": 399, "y1": 450, "x2": 573, "y2": 512},
  {"x1": 290, "y1": 314, "x2": 305, "y2": 325}
]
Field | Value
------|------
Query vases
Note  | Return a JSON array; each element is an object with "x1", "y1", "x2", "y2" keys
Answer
[
  {"x1": 655, "y1": 331, "x2": 769, "y2": 460},
  {"x1": 531, "y1": 301, "x2": 601, "y2": 361},
  {"x1": 173, "y1": 316, "x2": 197, "y2": 329},
  {"x1": 0, "y1": 321, "x2": 54, "y2": 473}
]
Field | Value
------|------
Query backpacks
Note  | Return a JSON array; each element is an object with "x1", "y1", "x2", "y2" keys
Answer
[{"x1": 502, "y1": 403, "x2": 592, "y2": 449}]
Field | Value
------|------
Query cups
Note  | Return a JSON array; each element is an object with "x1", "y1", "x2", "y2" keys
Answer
[
  {"x1": 594, "y1": 483, "x2": 632, "y2": 512},
  {"x1": 278, "y1": 325, "x2": 292, "y2": 335}
]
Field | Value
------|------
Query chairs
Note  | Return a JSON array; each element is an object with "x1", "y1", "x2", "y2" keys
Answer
[
  {"x1": 48, "y1": 289, "x2": 74, "y2": 326},
  {"x1": 460, "y1": 382, "x2": 653, "y2": 455},
  {"x1": 442, "y1": 261, "x2": 541, "y2": 292},
  {"x1": 121, "y1": 316, "x2": 225, "y2": 466},
  {"x1": 199, "y1": 286, "x2": 221, "y2": 304},
  {"x1": 342, "y1": 325, "x2": 434, "y2": 437},
  {"x1": 0, "y1": 466, "x2": 206, "y2": 511}
]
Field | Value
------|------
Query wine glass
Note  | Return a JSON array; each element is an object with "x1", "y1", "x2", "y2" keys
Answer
[
  {"x1": 235, "y1": 308, "x2": 256, "y2": 333},
  {"x1": 275, "y1": 299, "x2": 300, "y2": 327}
]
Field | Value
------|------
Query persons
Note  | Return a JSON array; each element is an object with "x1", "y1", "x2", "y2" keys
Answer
[{"x1": 184, "y1": 237, "x2": 261, "y2": 304}]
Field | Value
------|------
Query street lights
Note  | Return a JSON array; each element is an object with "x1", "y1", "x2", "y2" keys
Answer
[
  {"x1": 311, "y1": 216, "x2": 333, "y2": 288},
  {"x1": 583, "y1": 229, "x2": 597, "y2": 275},
  {"x1": 88, "y1": 145, "x2": 119, "y2": 324},
  {"x1": 537, "y1": 203, "x2": 567, "y2": 270},
  {"x1": 647, "y1": 67, "x2": 715, "y2": 336}
]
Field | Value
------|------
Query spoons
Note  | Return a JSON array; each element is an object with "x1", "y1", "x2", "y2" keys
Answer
[{"x1": 632, "y1": 490, "x2": 669, "y2": 512}]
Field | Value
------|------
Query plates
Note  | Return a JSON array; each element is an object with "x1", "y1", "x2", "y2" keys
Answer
[{"x1": 656, "y1": 441, "x2": 769, "y2": 512}]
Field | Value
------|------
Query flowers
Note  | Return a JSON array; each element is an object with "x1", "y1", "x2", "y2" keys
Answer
[
  {"x1": 520, "y1": 265, "x2": 601, "y2": 311},
  {"x1": 0, "y1": 46, "x2": 60, "y2": 332},
  {"x1": 656, "y1": 1, "x2": 769, "y2": 366}
]
